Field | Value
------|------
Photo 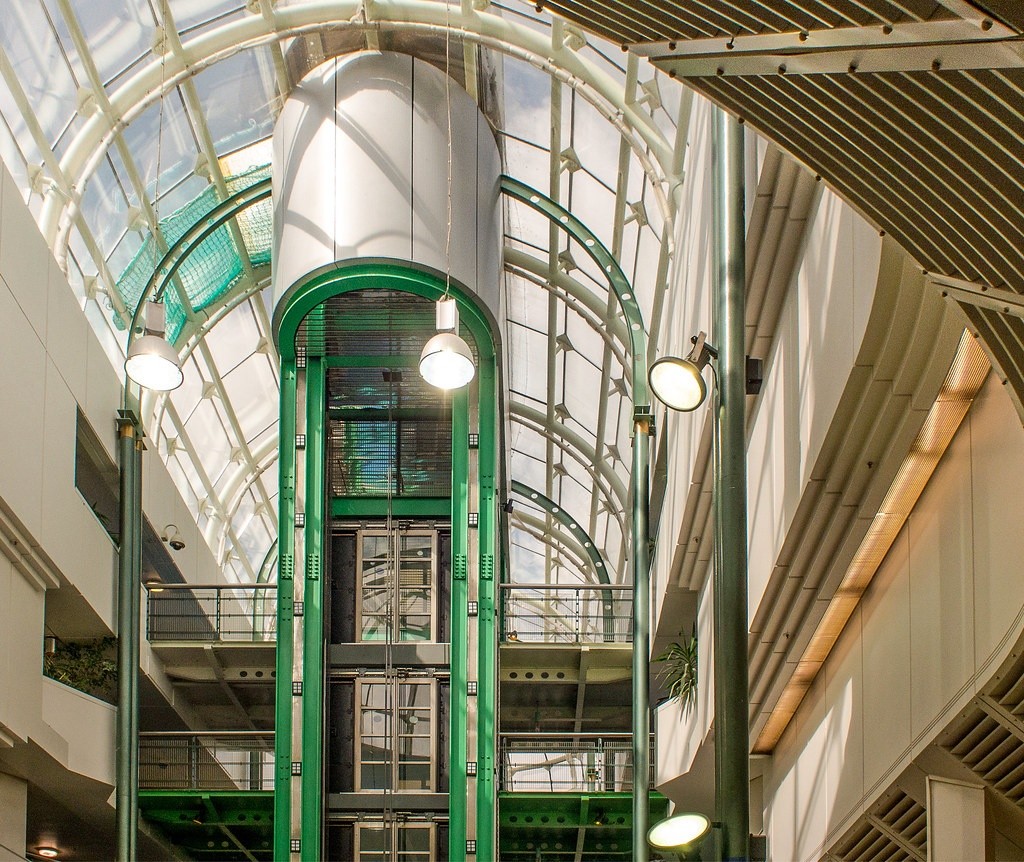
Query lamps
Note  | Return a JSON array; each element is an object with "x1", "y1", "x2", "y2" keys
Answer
[
  {"x1": 418, "y1": 299, "x2": 474, "y2": 390},
  {"x1": 123, "y1": 301, "x2": 183, "y2": 391},
  {"x1": 646, "y1": 331, "x2": 720, "y2": 412},
  {"x1": 645, "y1": 812, "x2": 713, "y2": 862},
  {"x1": 38, "y1": 848, "x2": 58, "y2": 857},
  {"x1": 145, "y1": 579, "x2": 165, "y2": 592}
]
